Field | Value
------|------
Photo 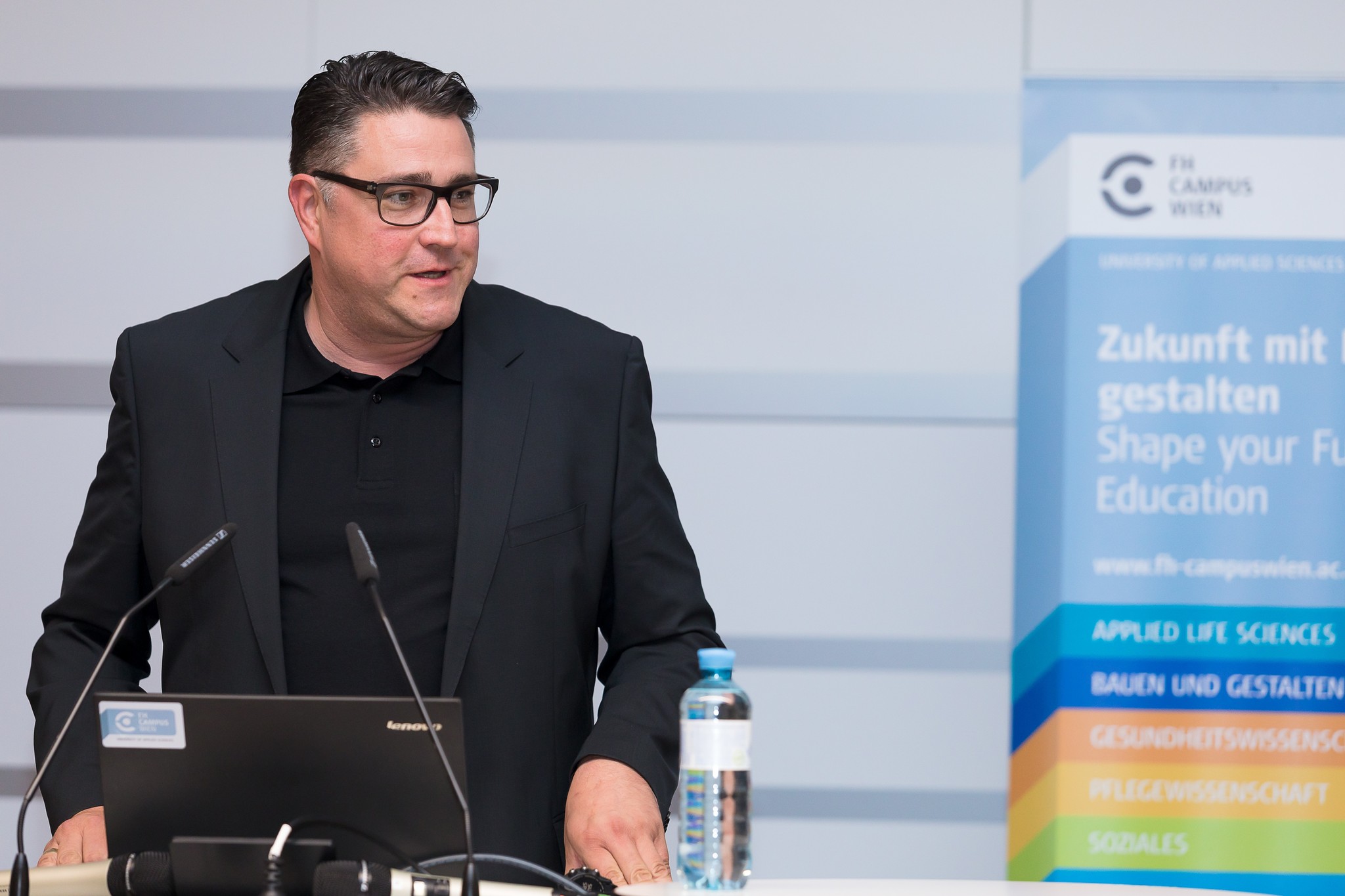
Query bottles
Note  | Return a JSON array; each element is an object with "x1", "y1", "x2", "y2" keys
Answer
[{"x1": 678, "y1": 646, "x2": 754, "y2": 891}]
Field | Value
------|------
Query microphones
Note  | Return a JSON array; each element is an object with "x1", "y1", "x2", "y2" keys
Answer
[
  {"x1": 314, "y1": 860, "x2": 575, "y2": 896},
  {"x1": 346, "y1": 521, "x2": 480, "y2": 896},
  {"x1": 0, "y1": 851, "x2": 174, "y2": 896},
  {"x1": 8, "y1": 523, "x2": 237, "y2": 896}
]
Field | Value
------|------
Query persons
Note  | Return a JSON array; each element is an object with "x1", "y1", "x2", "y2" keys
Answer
[{"x1": 25, "y1": 51, "x2": 726, "y2": 889}]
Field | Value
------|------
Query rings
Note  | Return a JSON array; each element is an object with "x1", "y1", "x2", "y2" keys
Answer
[{"x1": 41, "y1": 848, "x2": 58, "y2": 854}]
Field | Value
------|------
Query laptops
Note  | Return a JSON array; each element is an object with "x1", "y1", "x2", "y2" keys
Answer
[{"x1": 93, "y1": 691, "x2": 468, "y2": 896}]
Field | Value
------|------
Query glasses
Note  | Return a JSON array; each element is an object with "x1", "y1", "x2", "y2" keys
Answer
[{"x1": 311, "y1": 170, "x2": 499, "y2": 227}]
